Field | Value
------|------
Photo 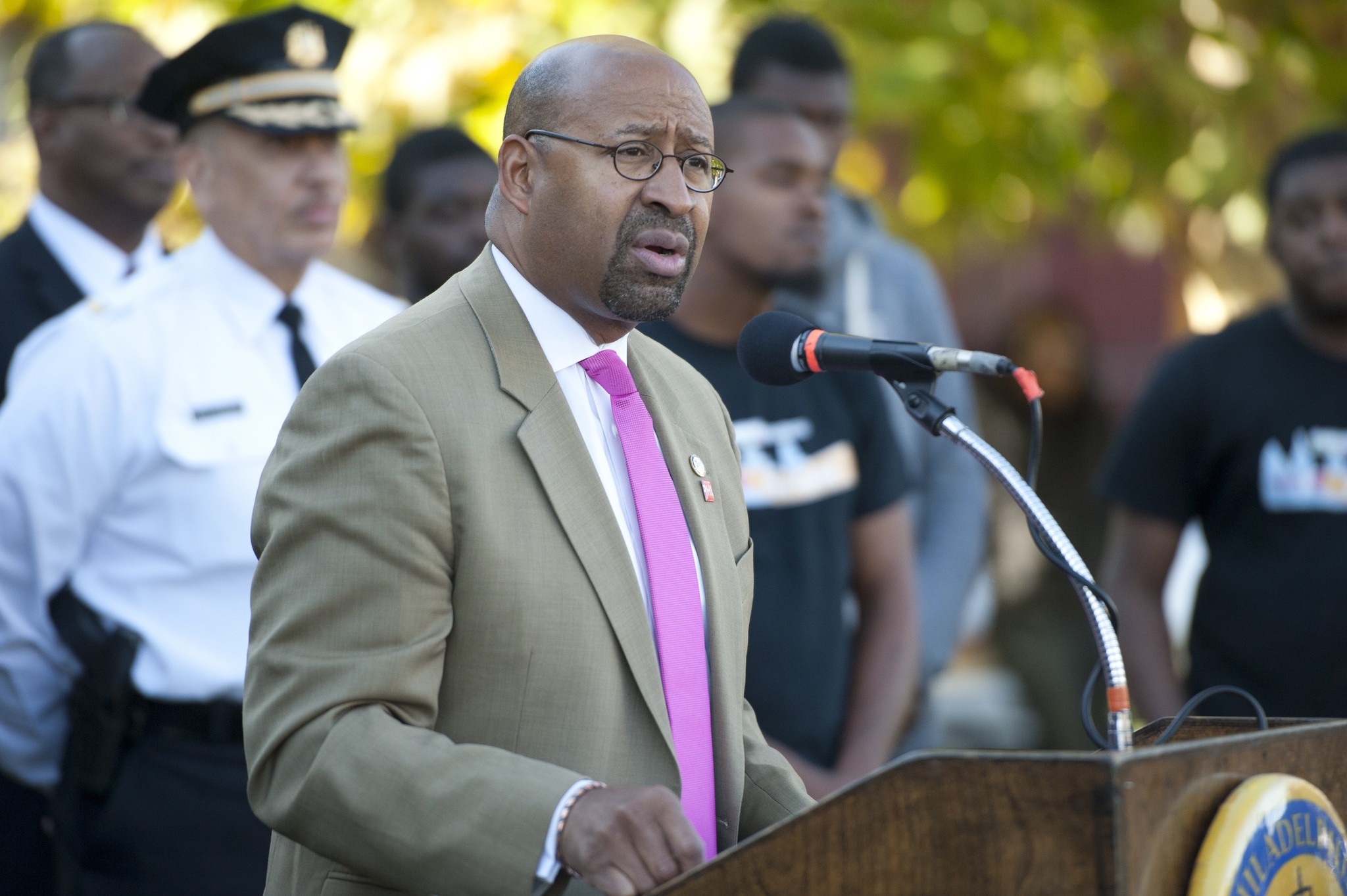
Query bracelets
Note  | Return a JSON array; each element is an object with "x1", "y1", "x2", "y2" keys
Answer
[{"x1": 555, "y1": 781, "x2": 607, "y2": 880}]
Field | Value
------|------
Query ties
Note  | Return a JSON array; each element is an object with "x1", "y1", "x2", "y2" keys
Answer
[
  {"x1": 278, "y1": 303, "x2": 315, "y2": 387},
  {"x1": 580, "y1": 349, "x2": 719, "y2": 861}
]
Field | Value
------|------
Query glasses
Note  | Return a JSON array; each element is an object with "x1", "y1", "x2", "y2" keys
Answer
[
  {"x1": 59, "y1": 90, "x2": 131, "y2": 123},
  {"x1": 523, "y1": 129, "x2": 734, "y2": 194}
]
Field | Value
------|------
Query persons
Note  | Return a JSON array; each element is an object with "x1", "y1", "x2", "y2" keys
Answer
[{"x1": 0, "y1": 5, "x2": 1347, "y2": 896}]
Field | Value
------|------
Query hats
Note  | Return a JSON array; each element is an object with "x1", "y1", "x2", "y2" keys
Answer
[{"x1": 134, "y1": 4, "x2": 356, "y2": 136}]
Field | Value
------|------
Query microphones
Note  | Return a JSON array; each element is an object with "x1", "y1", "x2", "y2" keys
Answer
[{"x1": 736, "y1": 308, "x2": 1016, "y2": 387}]
